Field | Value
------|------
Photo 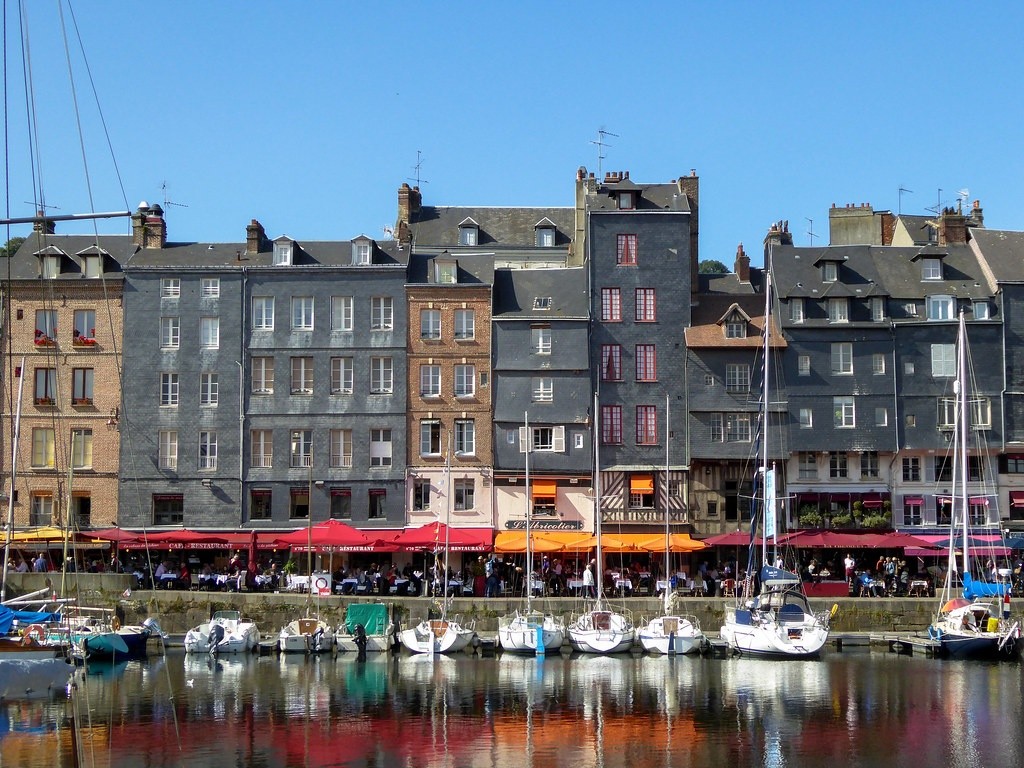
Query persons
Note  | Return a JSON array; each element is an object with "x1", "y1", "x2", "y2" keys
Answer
[
  {"x1": 66, "y1": 553, "x2": 1024, "y2": 598},
  {"x1": 7, "y1": 552, "x2": 62, "y2": 572}
]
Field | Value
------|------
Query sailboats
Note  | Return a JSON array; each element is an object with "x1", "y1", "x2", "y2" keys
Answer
[
  {"x1": 930, "y1": 308, "x2": 1024, "y2": 660},
  {"x1": 567, "y1": 391, "x2": 634, "y2": 655},
  {"x1": 634, "y1": 395, "x2": 710, "y2": 655},
  {"x1": 497, "y1": 409, "x2": 566, "y2": 654},
  {"x1": 720, "y1": 269, "x2": 832, "y2": 655},
  {"x1": 398, "y1": 428, "x2": 477, "y2": 653}
]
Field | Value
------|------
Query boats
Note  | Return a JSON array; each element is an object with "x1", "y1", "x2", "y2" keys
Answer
[
  {"x1": 0, "y1": 355, "x2": 170, "y2": 661},
  {"x1": 279, "y1": 588, "x2": 335, "y2": 652},
  {"x1": 335, "y1": 602, "x2": 396, "y2": 651},
  {"x1": 183, "y1": 611, "x2": 261, "y2": 653}
]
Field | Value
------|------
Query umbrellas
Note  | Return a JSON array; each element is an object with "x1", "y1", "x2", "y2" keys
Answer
[
  {"x1": 777, "y1": 531, "x2": 937, "y2": 547},
  {"x1": 495, "y1": 534, "x2": 565, "y2": 568},
  {"x1": 564, "y1": 535, "x2": 629, "y2": 548},
  {"x1": 72, "y1": 527, "x2": 140, "y2": 550},
  {"x1": 636, "y1": 533, "x2": 706, "y2": 574},
  {"x1": 701, "y1": 531, "x2": 774, "y2": 597},
  {"x1": 273, "y1": 517, "x2": 386, "y2": 574},
  {"x1": 385, "y1": 521, "x2": 485, "y2": 547},
  {"x1": 935, "y1": 535, "x2": 1024, "y2": 559},
  {"x1": 0, "y1": 526, "x2": 72, "y2": 555},
  {"x1": 139, "y1": 529, "x2": 228, "y2": 548}
]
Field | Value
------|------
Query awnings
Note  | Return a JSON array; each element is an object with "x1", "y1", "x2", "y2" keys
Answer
[
  {"x1": 602, "y1": 534, "x2": 691, "y2": 552},
  {"x1": 903, "y1": 535, "x2": 1011, "y2": 555},
  {"x1": 117, "y1": 532, "x2": 290, "y2": 549},
  {"x1": 863, "y1": 491, "x2": 1024, "y2": 507},
  {"x1": 291, "y1": 529, "x2": 403, "y2": 552},
  {"x1": 495, "y1": 531, "x2": 594, "y2": 553},
  {"x1": 631, "y1": 475, "x2": 653, "y2": 494},
  {"x1": 533, "y1": 480, "x2": 556, "y2": 497},
  {"x1": 404, "y1": 528, "x2": 494, "y2": 552}
]
